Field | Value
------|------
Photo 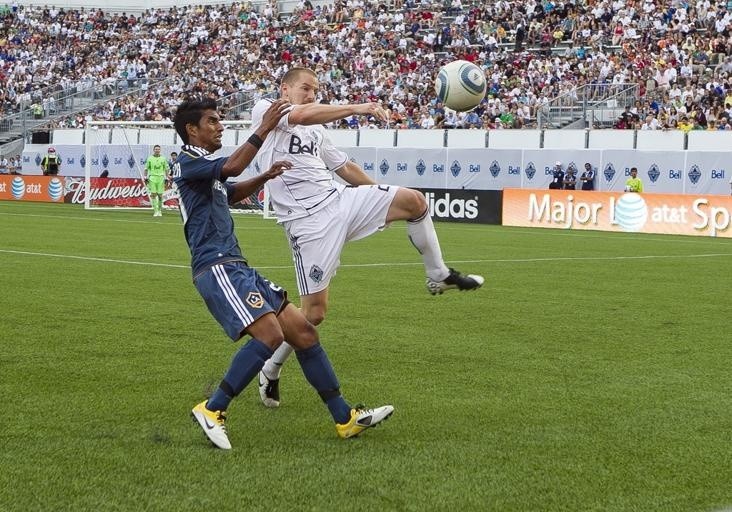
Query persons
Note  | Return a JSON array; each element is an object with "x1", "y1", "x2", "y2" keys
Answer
[
  {"x1": 250, "y1": 67, "x2": 487, "y2": 410},
  {"x1": 172, "y1": 98, "x2": 396, "y2": 451},
  {"x1": 1, "y1": 1, "x2": 732, "y2": 132},
  {"x1": 144, "y1": 144, "x2": 172, "y2": 217},
  {"x1": 173, "y1": 152, "x2": 177, "y2": 161},
  {"x1": 551, "y1": 162, "x2": 594, "y2": 190},
  {"x1": 0, "y1": 155, "x2": 21, "y2": 175},
  {"x1": 624, "y1": 168, "x2": 643, "y2": 193},
  {"x1": 41, "y1": 147, "x2": 57, "y2": 176}
]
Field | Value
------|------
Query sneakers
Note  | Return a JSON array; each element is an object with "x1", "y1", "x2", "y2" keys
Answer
[
  {"x1": 255, "y1": 367, "x2": 281, "y2": 408},
  {"x1": 335, "y1": 402, "x2": 395, "y2": 440},
  {"x1": 424, "y1": 267, "x2": 488, "y2": 295},
  {"x1": 188, "y1": 398, "x2": 233, "y2": 451},
  {"x1": 153, "y1": 211, "x2": 164, "y2": 217}
]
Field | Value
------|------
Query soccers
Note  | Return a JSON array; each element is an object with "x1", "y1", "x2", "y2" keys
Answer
[{"x1": 435, "y1": 60, "x2": 486, "y2": 112}]
